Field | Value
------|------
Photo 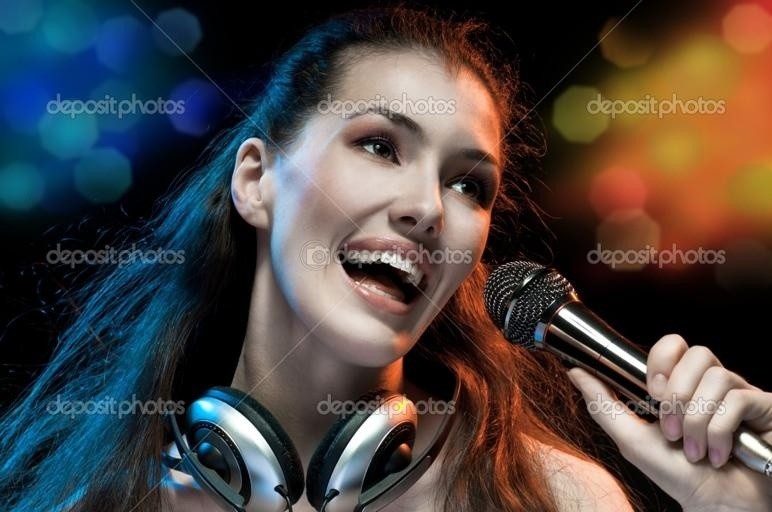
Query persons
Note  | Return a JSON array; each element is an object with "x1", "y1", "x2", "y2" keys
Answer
[{"x1": 2, "y1": 8, "x2": 772, "y2": 512}]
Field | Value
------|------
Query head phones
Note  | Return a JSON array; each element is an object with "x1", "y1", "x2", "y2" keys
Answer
[{"x1": 160, "y1": 347, "x2": 464, "y2": 512}]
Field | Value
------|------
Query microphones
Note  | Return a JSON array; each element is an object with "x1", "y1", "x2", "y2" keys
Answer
[{"x1": 482, "y1": 257, "x2": 772, "y2": 476}]
